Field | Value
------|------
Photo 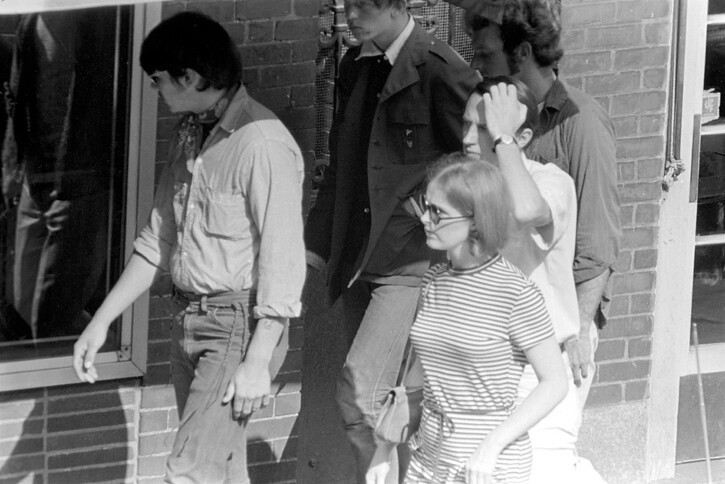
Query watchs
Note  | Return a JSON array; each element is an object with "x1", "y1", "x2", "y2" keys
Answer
[{"x1": 492, "y1": 134, "x2": 519, "y2": 154}]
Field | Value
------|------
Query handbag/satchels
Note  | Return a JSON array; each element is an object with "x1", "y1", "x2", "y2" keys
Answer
[{"x1": 375, "y1": 263, "x2": 449, "y2": 443}]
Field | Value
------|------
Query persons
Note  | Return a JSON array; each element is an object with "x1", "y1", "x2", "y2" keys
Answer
[
  {"x1": 306, "y1": 0, "x2": 619, "y2": 484},
  {"x1": 72, "y1": 9, "x2": 306, "y2": 484},
  {"x1": 4, "y1": 11, "x2": 121, "y2": 350}
]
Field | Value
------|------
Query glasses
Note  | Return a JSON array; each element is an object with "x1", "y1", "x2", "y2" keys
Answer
[{"x1": 419, "y1": 193, "x2": 474, "y2": 224}]
